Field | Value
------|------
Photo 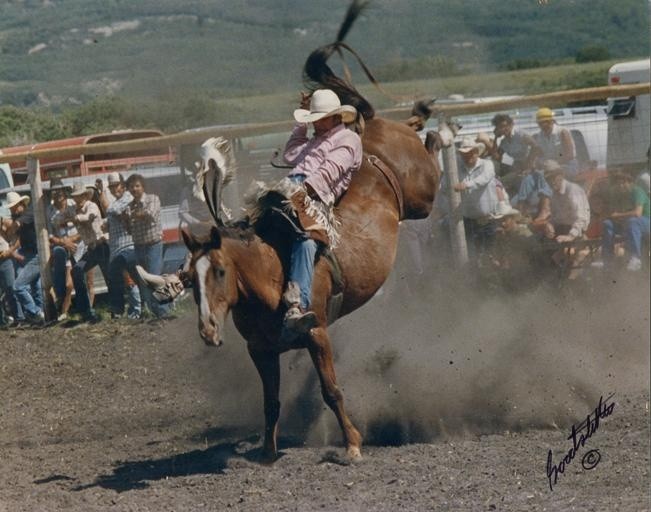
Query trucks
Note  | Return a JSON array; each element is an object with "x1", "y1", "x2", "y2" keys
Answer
[
  {"x1": 605, "y1": 59, "x2": 651, "y2": 173},
  {"x1": 0, "y1": 128, "x2": 173, "y2": 186}
]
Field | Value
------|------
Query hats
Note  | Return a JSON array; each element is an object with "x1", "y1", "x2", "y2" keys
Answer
[
  {"x1": 70, "y1": 179, "x2": 92, "y2": 196},
  {"x1": 457, "y1": 135, "x2": 487, "y2": 155},
  {"x1": 107, "y1": 171, "x2": 126, "y2": 188},
  {"x1": 489, "y1": 202, "x2": 522, "y2": 219},
  {"x1": 534, "y1": 107, "x2": 555, "y2": 123},
  {"x1": 3, "y1": 191, "x2": 30, "y2": 210},
  {"x1": 43, "y1": 177, "x2": 74, "y2": 196},
  {"x1": 292, "y1": 88, "x2": 358, "y2": 124},
  {"x1": 543, "y1": 159, "x2": 565, "y2": 178}
]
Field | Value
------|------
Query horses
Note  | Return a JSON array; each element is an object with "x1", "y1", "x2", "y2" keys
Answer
[{"x1": 179, "y1": 0, "x2": 462, "y2": 463}]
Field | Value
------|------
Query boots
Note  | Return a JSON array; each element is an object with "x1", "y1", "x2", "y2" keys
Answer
[
  {"x1": 133, "y1": 263, "x2": 184, "y2": 306},
  {"x1": 280, "y1": 307, "x2": 318, "y2": 342}
]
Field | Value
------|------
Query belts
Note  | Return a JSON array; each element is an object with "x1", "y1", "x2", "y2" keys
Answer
[
  {"x1": 134, "y1": 237, "x2": 164, "y2": 249},
  {"x1": 88, "y1": 236, "x2": 107, "y2": 251}
]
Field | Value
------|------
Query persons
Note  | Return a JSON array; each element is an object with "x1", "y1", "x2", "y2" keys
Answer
[
  {"x1": 5, "y1": 192, "x2": 45, "y2": 324},
  {"x1": 44, "y1": 177, "x2": 85, "y2": 308},
  {"x1": 516, "y1": 106, "x2": 576, "y2": 222},
  {"x1": 441, "y1": 136, "x2": 498, "y2": 261},
  {"x1": 95, "y1": 172, "x2": 140, "y2": 322},
  {"x1": 135, "y1": 89, "x2": 362, "y2": 343},
  {"x1": 53, "y1": 181, "x2": 94, "y2": 322},
  {"x1": 491, "y1": 114, "x2": 537, "y2": 184},
  {"x1": 51, "y1": 177, "x2": 109, "y2": 322},
  {"x1": 638, "y1": 147, "x2": 651, "y2": 192},
  {"x1": 591, "y1": 164, "x2": 650, "y2": 273},
  {"x1": 494, "y1": 177, "x2": 504, "y2": 202},
  {"x1": 0, "y1": 217, "x2": 26, "y2": 330},
  {"x1": 115, "y1": 174, "x2": 170, "y2": 318},
  {"x1": 490, "y1": 201, "x2": 532, "y2": 243},
  {"x1": 541, "y1": 158, "x2": 591, "y2": 245},
  {"x1": 510, "y1": 151, "x2": 543, "y2": 212},
  {"x1": 86, "y1": 178, "x2": 145, "y2": 320}
]
